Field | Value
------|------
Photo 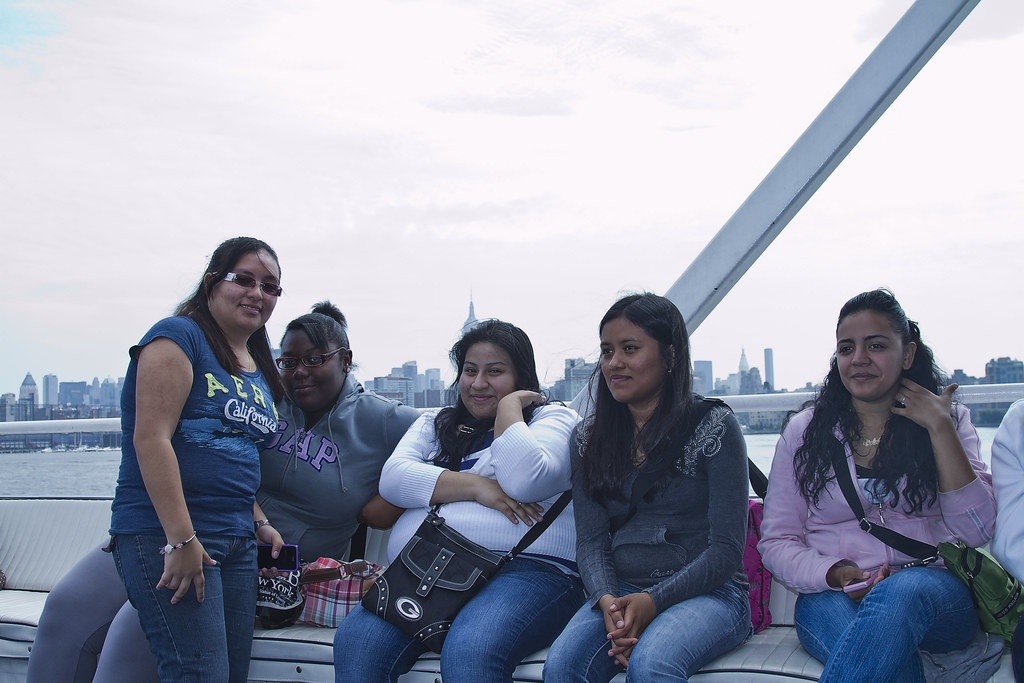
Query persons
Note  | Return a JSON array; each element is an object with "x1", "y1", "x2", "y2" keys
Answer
[
  {"x1": 27, "y1": 302, "x2": 422, "y2": 683},
  {"x1": 541, "y1": 293, "x2": 752, "y2": 680},
  {"x1": 758, "y1": 289, "x2": 996, "y2": 683},
  {"x1": 109, "y1": 235, "x2": 280, "y2": 683},
  {"x1": 333, "y1": 319, "x2": 584, "y2": 683},
  {"x1": 992, "y1": 399, "x2": 1024, "y2": 683}
]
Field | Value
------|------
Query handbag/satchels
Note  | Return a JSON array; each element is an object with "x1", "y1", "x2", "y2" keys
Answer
[
  {"x1": 744, "y1": 499, "x2": 774, "y2": 632},
  {"x1": 297, "y1": 557, "x2": 388, "y2": 628},
  {"x1": 938, "y1": 542, "x2": 1024, "y2": 641},
  {"x1": 257, "y1": 560, "x2": 309, "y2": 628},
  {"x1": 361, "y1": 511, "x2": 511, "y2": 655}
]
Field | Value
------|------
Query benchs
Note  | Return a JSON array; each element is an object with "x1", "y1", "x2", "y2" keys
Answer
[{"x1": 0, "y1": 499, "x2": 1015, "y2": 683}]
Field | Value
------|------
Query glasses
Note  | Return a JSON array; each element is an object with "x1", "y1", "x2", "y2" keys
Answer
[
  {"x1": 275, "y1": 346, "x2": 347, "y2": 370},
  {"x1": 213, "y1": 272, "x2": 282, "y2": 297}
]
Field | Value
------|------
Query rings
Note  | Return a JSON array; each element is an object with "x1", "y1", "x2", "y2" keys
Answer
[
  {"x1": 517, "y1": 500, "x2": 522, "y2": 505},
  {"x1": 900, "y1": 397, "x2": 906, "y2": 405}
]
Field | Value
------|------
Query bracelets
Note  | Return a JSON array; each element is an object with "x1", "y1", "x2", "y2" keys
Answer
[
  {"x1": 253, "y1": 520, "x2": 272, "y2": 531},
  {"x1": 160, "y1": 531, "x2": 196, "y2": 556}
]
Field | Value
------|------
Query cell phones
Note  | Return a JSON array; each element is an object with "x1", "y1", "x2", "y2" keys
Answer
[
  {"x1": 843, "y1": 570, "x2": 880, "y2": 593},
  {"x1": 257, "y1": 544, "x2": 299, "y2": 572}
]
